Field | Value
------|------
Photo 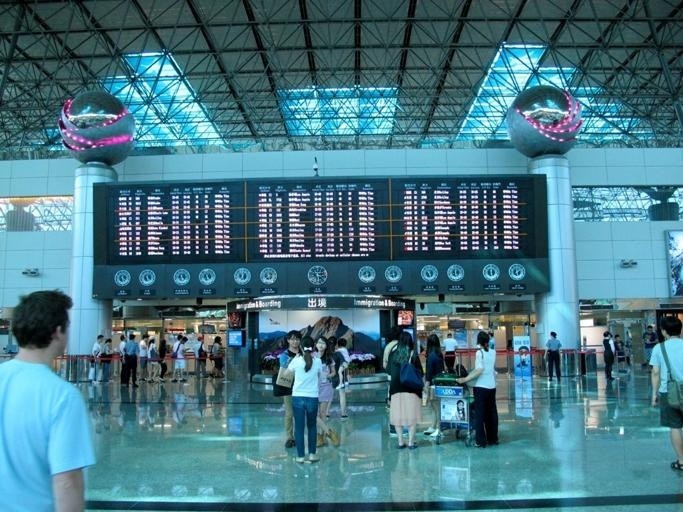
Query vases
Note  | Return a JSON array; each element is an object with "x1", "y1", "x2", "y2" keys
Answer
[
  {"x1": 352, "y1": 367, "x2": 375, "y2": 375},
  {"x1": 260, "y1": 370, "x2": 280, "y2": 376}
]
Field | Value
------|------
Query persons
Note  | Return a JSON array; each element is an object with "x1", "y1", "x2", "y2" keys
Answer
[
  {"x1": 0, "y1": 288, "x2": 95, "y2": 511},
  {"x1": 382, "y1": 328, "x2": 499, "y2": 448},
  {"x1": 648, "y1": 315, "x2": 683, "y2": 475},
  {"x1": 88, "y1": 332, "x2": 225, "y2": 388},
  {"x1": 275, "y1": 330, "x2": 350, "y2": 465},
  {"x1": 602, "y1": 332, "x2": 625, "y2": 379},
  {"x1": 544, "y1": 332, "x2": 563, "y2": 382},
  {"x1": 87, "y1": 383, "x2": 223, "y2": 432},
  {"x1": 548, "y1": 382, "x2": 564, "y2": 429}
]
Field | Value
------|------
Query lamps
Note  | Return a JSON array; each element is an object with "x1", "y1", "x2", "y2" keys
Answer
[
  {"x1": 22, "y1": 268, "x2": 39, "y2": 278},
  {"x1": 621, "y1": 259, "x2": 638, "y2": 267}
]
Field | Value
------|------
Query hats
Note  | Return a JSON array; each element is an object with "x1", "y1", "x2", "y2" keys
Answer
[{"x1": 149, "y1": 336, "x2": 154, "y2": 340}]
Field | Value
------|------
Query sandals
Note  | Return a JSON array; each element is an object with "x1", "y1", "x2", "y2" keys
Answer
[{"x1": 671, "y1": 460, "x2": 683, "y2": 471}]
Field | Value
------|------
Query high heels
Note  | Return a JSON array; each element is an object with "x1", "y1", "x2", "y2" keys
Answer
[{"x1": 474, "y1": 441, "x2": 499, "y2": 448}]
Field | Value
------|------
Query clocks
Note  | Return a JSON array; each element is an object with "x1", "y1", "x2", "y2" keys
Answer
[
  {"x1": 198, "y1": 268, "x2": 217, "y2": 287},
  {"x1": 307, "y1": 265, "x2": 329, "y2": 286},
  {"x1": 258, "y1": 267, "x2": 278, "y2": 286},
  {"x1": 508, "y1": 264, "x2": 526, "y2": 281},
  {"x1": 232, "y1": 267, "x2": 252, "y2": 286},
  {"x1": 446, "y1": 264, "x2": 466, "y2": 283},
  {"x1": 172, "y1": 268, "x2": 191, "y2": 287},
  {"x1": 138, "y1": 268, "x2": 157, "y2": 287},
  {"x1": 420, "y1": 265, "x2": 439, "y2": 284},
  {"x1": 384, "y1": 265, "x2": 403, "y2": 285},
  {"x1": 358, "y1": 265, "x2": 377, "y2": 284},
  {"x1": 115, "y1": 269, "x2": 132, "y2": 287},
  {"x1": 481, "y1": 264, "x2": 501, "y2": 282}
]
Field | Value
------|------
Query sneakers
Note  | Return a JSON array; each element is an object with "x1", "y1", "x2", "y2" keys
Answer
[
  {"x1": 336, "y1": 381, "x2": 348, "y2": 389},
  {"x1": 389, "y1": 426, "x2": 444, "y2": 449},
  {"x1": 293, "y1": 453, "x2": 319, "y2": 462}
]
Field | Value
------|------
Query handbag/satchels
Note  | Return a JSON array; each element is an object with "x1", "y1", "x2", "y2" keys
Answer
[
  {"x1": 198, "y1": 348, "x2": 207, "y2": 361},
  {"x1": 399, "y1": 350, "x2": 424, "y2": 388},
  {"x1": 101, "y1": 353, "x2": 112, "y2": 363},
  {"x1": 151, "y1": 349, "x2": 159, "y2": 360},
  {"x1": 467, "y1": 369, "x2": 479, "y2": 387},
  {"x1": 667, "y1": 380, "x2": 683, "y2": 411},
  {"x1": 272, "y1": 369, "x2": 295, "y2": 396},
  {"x1": 210, "y1": 354, "x2": 215, "y2": 360},
  {"x1": 171, "y1": 352, "x2": 177, "y2": 358}
]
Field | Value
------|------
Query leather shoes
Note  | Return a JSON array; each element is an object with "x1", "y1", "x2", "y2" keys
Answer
[{"x1": 286, "y1": 439, "x2": 295, "y2": 447}]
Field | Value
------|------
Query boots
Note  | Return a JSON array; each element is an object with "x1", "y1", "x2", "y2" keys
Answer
[{"x1": 316, "y1": 428, "x2": 340, "y2": 447}]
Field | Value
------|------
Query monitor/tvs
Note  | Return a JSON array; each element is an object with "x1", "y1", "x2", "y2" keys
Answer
[
  {"x1": 404, "y1": 328, "x2": 413, "y2": 340},
  {"x1": 227, "y1": 330, "x2": 245, "y2": 347}
]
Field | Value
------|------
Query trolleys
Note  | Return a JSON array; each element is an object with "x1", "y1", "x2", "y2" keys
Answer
[
  {"x1": 431, "y1": 377, "x2": 476, "y2": 447},
  {"x1": 432, "y1": 446, "x2": 486, "y2": 503}
]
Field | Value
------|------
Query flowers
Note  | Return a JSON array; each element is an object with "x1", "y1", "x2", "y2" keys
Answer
[
  {"x1": 261, "y1": 350, "x2": 282, "y2": 369},
  {"x1": 348, "y1": 353, "x2": 381, "y2": 370}
]
